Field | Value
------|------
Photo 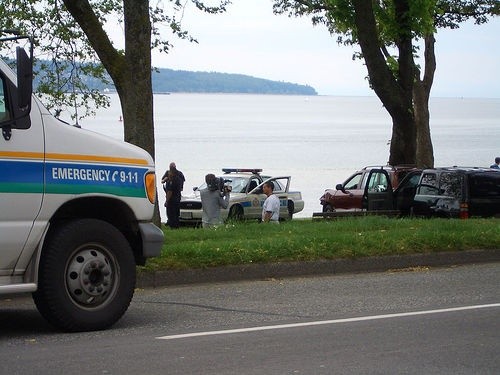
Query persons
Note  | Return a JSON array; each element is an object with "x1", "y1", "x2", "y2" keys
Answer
[
  {"x1": 262, "y1": 182, "x2": 280, "y2": 225},
  {"x1": 490, "y1": 157, "x2": 500, "y2": 168},
  {"x1": 200, "y1": 174, "x2": 230, "y2": 229},
  {"x1": 161, "y1": 162, "x2": 185, "y2": 230}
]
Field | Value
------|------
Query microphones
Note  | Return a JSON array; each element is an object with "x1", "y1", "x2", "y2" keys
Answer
[{"x1": 225, "y1": 180, "x2": 232, "y2": 183}]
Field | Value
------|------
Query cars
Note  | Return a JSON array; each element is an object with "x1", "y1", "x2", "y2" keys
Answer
[{"x1": 179, "y1": 168, "x2": 304, "y2": 225}]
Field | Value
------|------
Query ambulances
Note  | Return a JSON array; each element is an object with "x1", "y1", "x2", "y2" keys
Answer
[{"x1": 0, "y1": 47, "x2": 164, "y2": 333}]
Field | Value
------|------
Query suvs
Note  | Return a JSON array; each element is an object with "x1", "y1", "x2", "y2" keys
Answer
[
  {"x1": 362, "y1": 168, "x2": 500, "y2": 220},
  {"x1": 320, "y1": 166, "x2": 427, "y2": 218}
]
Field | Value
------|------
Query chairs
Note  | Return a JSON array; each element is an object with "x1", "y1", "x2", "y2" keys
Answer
[{"x1": 244, "y1": 181, "x2": 257, "y2": 193}]
